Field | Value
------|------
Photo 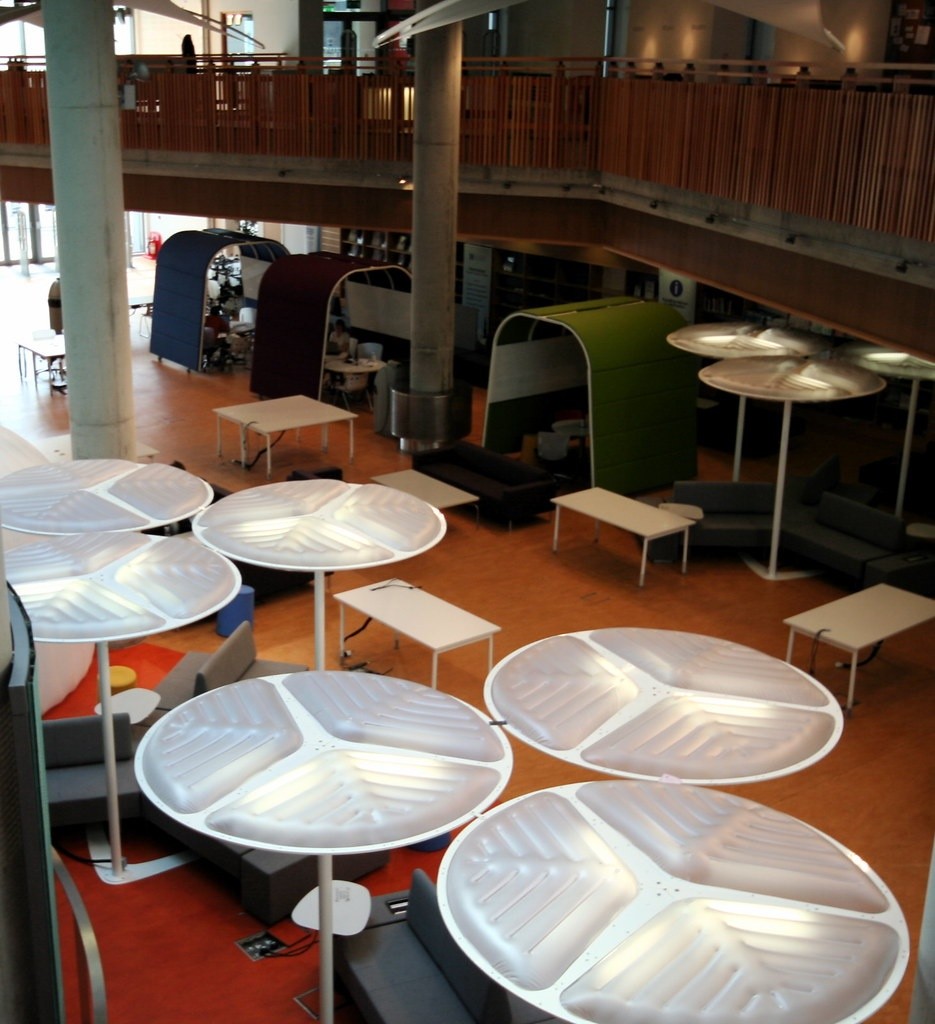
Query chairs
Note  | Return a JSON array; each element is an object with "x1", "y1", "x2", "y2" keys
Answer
[
  {"x1": 238, "y1": 306, "x2": 257, "y2": 323},
  {"x1": 348, "y1": 338, "x2": 358, "y2": 356},
  {"x1": 203, "y1": 327, "x2": 223, "y2": 372},
  {"x1": 358, "y1": 343, "x2": 383, "y2": 359}
]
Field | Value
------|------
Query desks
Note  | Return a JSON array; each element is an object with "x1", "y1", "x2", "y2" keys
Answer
[
  {"x1": 17, "y1": 333, "x2": 66, "y2": 398},
  {"x1": 369, "y1": 469, "x2": 480, "y2": 511},
  {"x1": 551, "y1": 418, "x2": 588, "y2": 445},
  {"x1": 549, "y1": 486, "x2": 696, "y2": 587},
  {"x1": 212, "y1": 394, "x2": 359, "y2": 479},
  {"x1": 783, "y1": 583, "x2": 935, "y2": 716},
  {"x1": 228, "y1": 320, "x2": 256, "y2": 334},
  {"x1": 331, "y1": 577, "x2": 501, "y2": 694},
  {"x1": 324, "y1": 356, "x2": 388, "y2": 414}
]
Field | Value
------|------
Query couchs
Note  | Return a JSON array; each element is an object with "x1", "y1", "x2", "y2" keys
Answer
[
  {"x1": 672, "y1": 479, "x2": 929, "y2": 589},
  {"x1": 333, "y1": 868, "x2": 571, "y2": 1024},
  {"x1": 142, "y1": 803, "x2": 392, "y2": 923},
  {"x1": 411, "y1": 440, "x2": 559, "y2": 524},
  {"x1": 40, "y1": 713, "x2": 141, "y2": 832},
  {"x1": 128, "y1": 621, "x2": 309, "y2": 754}
]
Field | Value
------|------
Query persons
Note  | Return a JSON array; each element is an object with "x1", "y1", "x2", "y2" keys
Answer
[
  {"x1": 219, "y1": 285, "x2": 244, "y2": 323},
  {"x1": 204, "y1": 306, "x2": 230, "y2": 375},
  {"x1": 329, "y1": 319, "x2": 351, "y2": 387}
]
